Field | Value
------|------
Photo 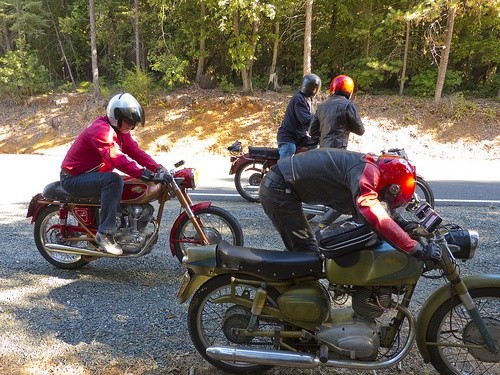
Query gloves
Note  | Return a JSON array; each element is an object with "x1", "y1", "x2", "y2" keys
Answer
[
  {"x1": 413, "y1": 245, "x2": 442, "y2": 265},
  {"x1": 141, "y1": 169, "x2": 171, "y2": 185}
]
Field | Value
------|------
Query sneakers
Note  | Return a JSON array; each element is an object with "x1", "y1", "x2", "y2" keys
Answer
[{"x1": 94, "y1": 231, "x2": 123, "y2": 255}]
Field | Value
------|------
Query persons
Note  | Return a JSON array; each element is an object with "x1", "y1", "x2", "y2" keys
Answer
[
  {"x1": 60, "y1": 92, "x2": 174, "y2": 256},
  {"x1": 309, "y1": 75, "x2": 365, "y2": 150},
  {"x1": 277, "y1": 74, "x2": 321, "y2": 160},
  {"x1": 258, "y1": 147, "x2": 442, "y2": 264}
]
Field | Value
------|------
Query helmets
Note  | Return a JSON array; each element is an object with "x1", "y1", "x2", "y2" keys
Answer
[
  {"x1": 377, "y1": 153, "x2": 416, "y2": 208},
  {"x1": 106, "y1": 92, "x2": 143, "y2": 128},
  {"x1": 329, "y1": 75, "x2": 355, "y2": 99},
  {"x1": 300, "y1": 74, "x2": 321, "y2": 95}
]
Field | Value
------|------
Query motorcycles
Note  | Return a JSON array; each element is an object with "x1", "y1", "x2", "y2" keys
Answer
[
  {"x1": 367, "y1": 146, "x2": 435, "y2": 211},
  {"x1": 26, "y1": 160, "x2": 244, "y2": 271},
  {"x1": 176, "y1": 189, "x2": 499, "y2": 375},
  {"x1": 225, "y1": 140, "x2": 320, "y2": 203}
]
